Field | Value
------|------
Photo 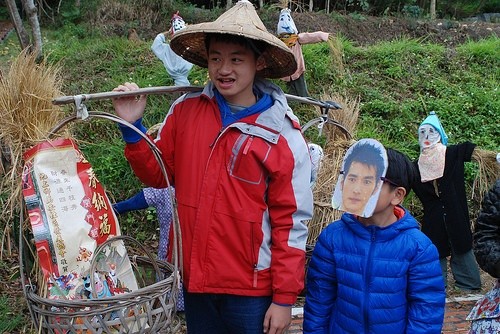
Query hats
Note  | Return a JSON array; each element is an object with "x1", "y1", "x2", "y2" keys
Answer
[{"x1": 168, "y1": 1, "x2": 297, "y2": 80}]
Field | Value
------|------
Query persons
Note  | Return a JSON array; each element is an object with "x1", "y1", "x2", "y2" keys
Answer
[
  {"x1": 410, "y1": 111, "x2": 500, "y2": 291},
  {"x1": 152, "y1": 14, "x2": 194, "y2": 86},
  {"x1": 336, "y1": 141, "x2": 384, "y2": 217},
  {"x1": 276, "y1": 9, "x2": 329, "y2": 99},
  {"x1": 303, "y1": 148, "x2": 446, "y2": 334},
  {"x1": 112, "y1": 0, "x2": 315, "y2": 334},
  {"x1": 474, "y1": 178, "x2": 500, "y2": 278},
  {"x1": 109, "y1": 185, "x2": 184, "y2": 312}
]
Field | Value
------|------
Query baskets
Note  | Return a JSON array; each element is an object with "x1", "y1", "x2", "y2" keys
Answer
[{"x1": 17, "y1": 111, "x2": 187, "y2": 334}]
